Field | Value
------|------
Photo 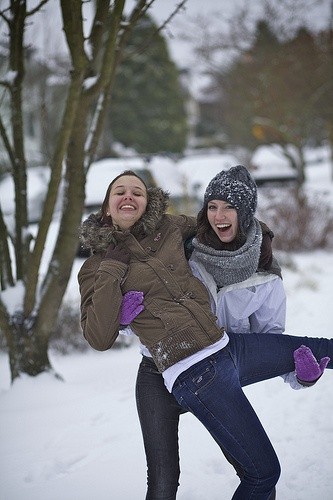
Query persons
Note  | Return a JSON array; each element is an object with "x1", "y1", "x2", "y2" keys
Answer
[
  {"x1": 77, "y1": 170, "x2": 333, "y2": 500},
  {"x1": 117, "y1": 165, "x2": 329, "y2": 500}
]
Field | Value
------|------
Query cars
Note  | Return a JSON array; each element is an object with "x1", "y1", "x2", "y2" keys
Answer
[{"x1": 251, "y1": 153, "x2": 299, "y2": 187}]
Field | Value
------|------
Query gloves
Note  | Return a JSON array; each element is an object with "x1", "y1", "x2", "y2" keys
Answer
[
  {"x1": 293, "y1": 345, "x2": 330, "y2": 387},
  {"x1": 120, "y1": 290, "x2": 143, "y2": 325},
  {"x1": 259, "y1": 237, "x2": 272, "y2": 270},
  {"x1": 104, "y1": 242, "x2": 129, "y2": 265}
]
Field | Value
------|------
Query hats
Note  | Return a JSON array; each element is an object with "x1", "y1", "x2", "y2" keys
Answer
[{"x1": 203, "y1": 164, "x2": 257, "y2": 236}]
